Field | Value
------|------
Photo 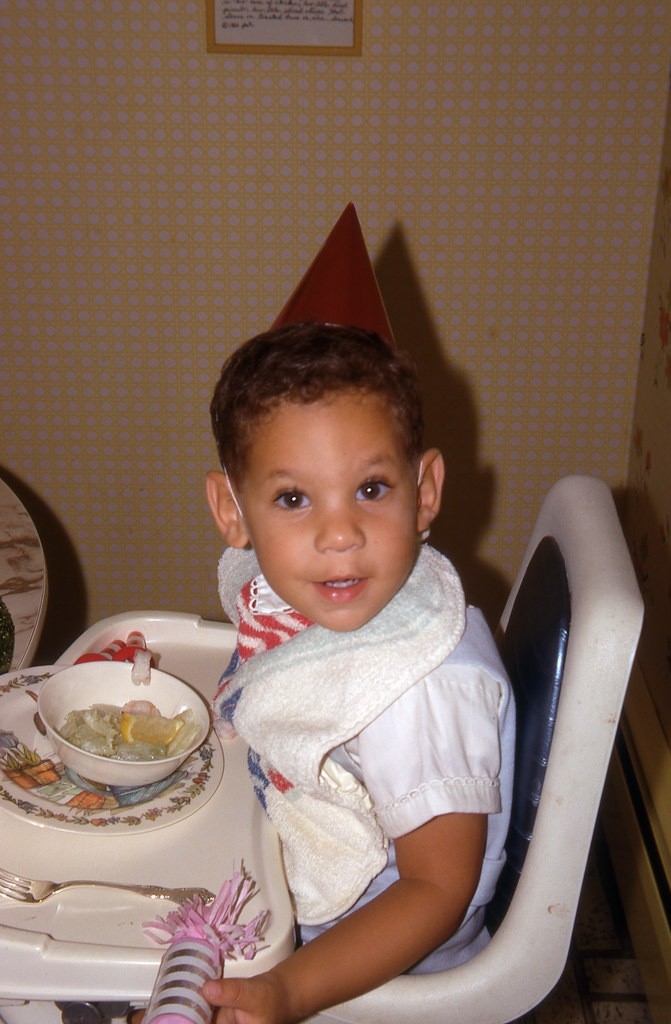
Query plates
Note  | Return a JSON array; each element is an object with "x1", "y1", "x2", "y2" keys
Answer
[{"x1": 0, "y1": 664, "x2": 225, "y2": 837}]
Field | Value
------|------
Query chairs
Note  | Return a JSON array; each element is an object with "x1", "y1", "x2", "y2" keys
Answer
[{"x1": 301, "y1": 476, "x2": 645, "y2": 1023}]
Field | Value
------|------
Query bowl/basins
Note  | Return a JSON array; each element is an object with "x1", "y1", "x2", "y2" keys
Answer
[{"x1": 36, "y1": 660, "x2": 210, "y2": 786}]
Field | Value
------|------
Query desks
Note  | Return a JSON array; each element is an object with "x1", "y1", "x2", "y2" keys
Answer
[{"x1": 0, "y1": 606, "x2": 296, "y2": 1006}]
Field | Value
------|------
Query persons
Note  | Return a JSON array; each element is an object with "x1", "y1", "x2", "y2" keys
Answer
[{"x1": 203, "y1": 324, "x2": 517, "y2": 1024}]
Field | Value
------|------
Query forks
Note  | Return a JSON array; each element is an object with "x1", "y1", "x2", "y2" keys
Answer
[{"x1": 0, "y1": 869, "x2": 216, "y2": 907}]
